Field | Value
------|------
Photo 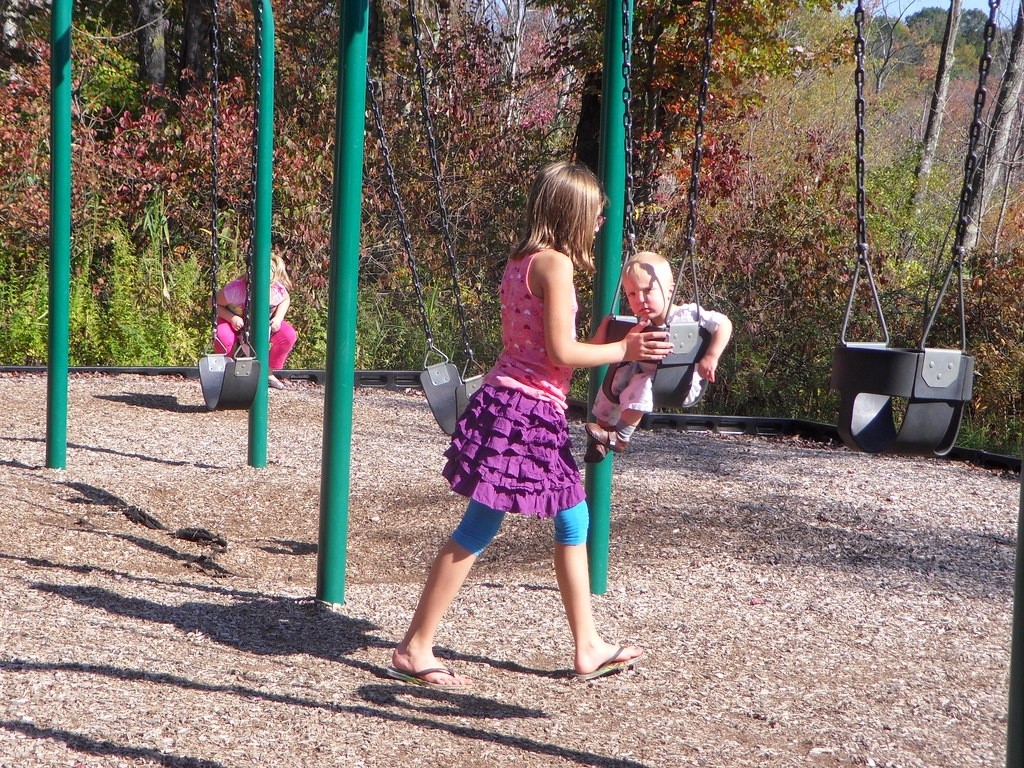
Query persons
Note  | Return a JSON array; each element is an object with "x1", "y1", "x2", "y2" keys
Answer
[
  {"x1": 206, "y1": 251, "x2": 299, "y2": 390},
  {"x1": 582, "y1": 251, "x2": 733, "y2": 464},
  {"x1": 384, "y1": 159, "x2": 649, "y2": 690}
]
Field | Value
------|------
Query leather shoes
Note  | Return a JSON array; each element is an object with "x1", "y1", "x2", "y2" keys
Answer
[
  {"x1": 584, "y1": 423, "x2": 628, "y2": 454},
  {"x1": 585, "y1": 441, "x2": 607, "y2": 464}
]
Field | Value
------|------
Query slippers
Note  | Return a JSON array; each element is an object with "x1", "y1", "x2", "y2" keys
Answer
[
  {"x1": 382, "y1": 666, "x2": 471, "y2": 690},
  {"x1": 578, "y1": 643, "x2": 647, "y2": 683}
]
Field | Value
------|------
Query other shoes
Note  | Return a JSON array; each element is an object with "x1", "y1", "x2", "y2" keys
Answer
[{"x1": 268, "y1": 376, "x2": 284, "y2": 390}]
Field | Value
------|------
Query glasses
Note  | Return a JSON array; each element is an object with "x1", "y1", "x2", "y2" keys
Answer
[{"x1": 596, "y1": 214, "x2": 606, "y2": 227}]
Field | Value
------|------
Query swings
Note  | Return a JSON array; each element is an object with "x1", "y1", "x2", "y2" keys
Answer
[
  {"x1": 829, "y1": 0, "x2": 1000, "y2": 459},
  {"x1": 600, "y1": 1, "x2": 720, "y2": 411},
  {"x1": 365, "y1": 0, "x2": 489, "y2": 437},
  {"x1": 197, "y1": 0, "x2": 261, "y2": 412}
]
